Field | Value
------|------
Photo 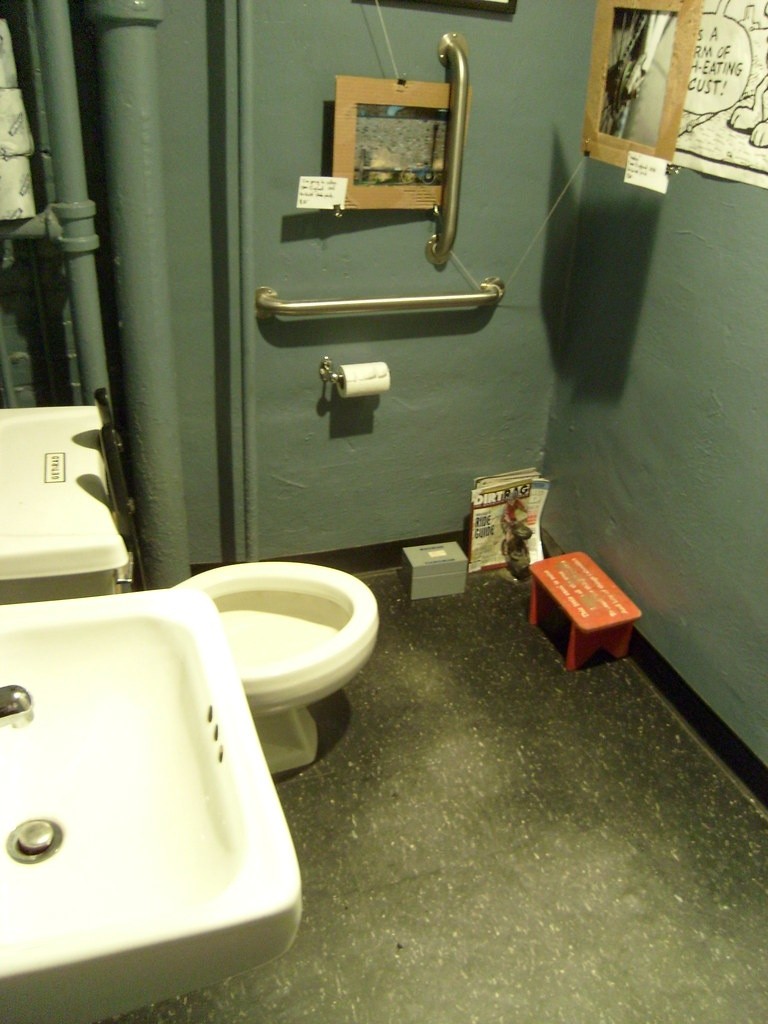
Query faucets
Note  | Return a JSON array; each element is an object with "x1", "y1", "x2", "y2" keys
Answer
[{"x1": 0, "y1": 684, "x2": 34, "y2": 730}]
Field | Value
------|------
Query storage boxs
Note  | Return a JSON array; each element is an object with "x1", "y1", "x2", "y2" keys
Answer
[{"x1": 401, "y1": 541, "x2": 468, "y2": 601}]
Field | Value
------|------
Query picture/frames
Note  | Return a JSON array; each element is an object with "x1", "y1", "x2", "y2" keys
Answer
[
  {"x1": 581, "y1": 0, "x2": 703, "y2": 172},
  {"x1": 331, "y1": 74, "x2": 472, "y2": 209}
]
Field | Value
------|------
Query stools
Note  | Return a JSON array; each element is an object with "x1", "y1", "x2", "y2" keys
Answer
[{"x1": 529, "y1": 549, "x2": 644, "y2": 670}]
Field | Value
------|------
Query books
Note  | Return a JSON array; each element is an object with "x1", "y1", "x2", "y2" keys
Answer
[{"x1": 468, "y1": 468, "x2": 550, "y2": 572}]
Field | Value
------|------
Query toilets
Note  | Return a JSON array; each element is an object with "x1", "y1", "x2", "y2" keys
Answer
[{"x1": 168, "y1": 561, "x2": 381, "y2": 777}]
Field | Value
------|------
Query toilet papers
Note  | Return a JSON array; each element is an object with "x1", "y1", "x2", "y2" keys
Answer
[{"x1": 337, "y1": 361, "x2": 390, "y2": 398}]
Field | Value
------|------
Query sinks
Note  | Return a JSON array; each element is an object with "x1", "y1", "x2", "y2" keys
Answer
[{"x1": 0, "y1": 590, "x2": 305, "y2": 1024}]
